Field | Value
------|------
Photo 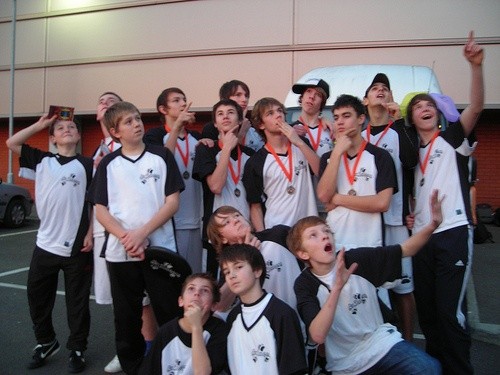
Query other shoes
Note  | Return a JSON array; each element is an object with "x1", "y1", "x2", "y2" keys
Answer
[{"x1": 104, "y1": 356, "x2": 123, "y2": 373}]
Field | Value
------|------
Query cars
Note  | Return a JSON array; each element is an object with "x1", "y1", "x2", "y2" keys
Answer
[{"x1": 0, "y1": 179, "x2": 35, "y2": 229}]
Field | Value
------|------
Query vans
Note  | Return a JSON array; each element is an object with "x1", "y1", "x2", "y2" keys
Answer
[{"x1": 283, "y1": 64, "x2": 448, "y2": 131}]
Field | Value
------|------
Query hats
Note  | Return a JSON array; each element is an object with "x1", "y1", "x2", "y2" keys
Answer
[
  {"x1": 365, "y1": 73, "x2": 390, "y2": 97},
  {"x1": 293, "y1": 79, "x2": 329, "y2": 97}
]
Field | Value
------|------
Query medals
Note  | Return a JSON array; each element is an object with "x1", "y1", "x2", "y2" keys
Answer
[
  {"x1": 183, "y1": 170, "x2": 190, "y2": 181},
  {"x1": 235, "y1": 187, "x2": 240, "y2": 196},
  {"x1": 287, "y1": 186, "x2": 294, "y2": 193},
  {"x1": 348, "y1": 190, "x2": 356, "y2": 196},
  {"x1": 419, "y1": 178, "x2": 424, "y2": 186}
]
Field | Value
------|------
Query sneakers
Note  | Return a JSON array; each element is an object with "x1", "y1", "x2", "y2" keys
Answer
[
  {"x1": 66, "y1": 350, "x2": 85, "y2": 372},
  {"x1": 27, "y1": 339, "x2": 60, "y2": 369}
]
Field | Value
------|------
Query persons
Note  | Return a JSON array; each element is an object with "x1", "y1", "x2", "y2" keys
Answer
[{"x1": 5, "y1": 30, "x2": 486, "y2": 375}]
